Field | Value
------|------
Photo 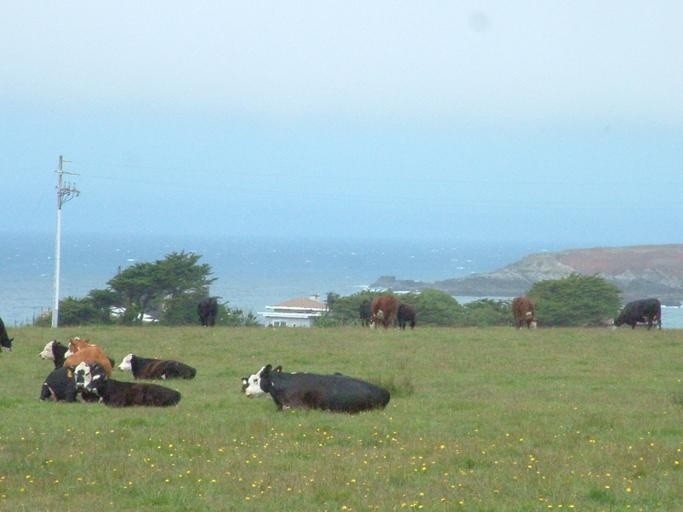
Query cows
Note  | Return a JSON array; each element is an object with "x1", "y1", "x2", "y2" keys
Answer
[
  {"x1": 358, "y1": 295, "x2": 418, "y2": 330},
  {"x1": 611, "y1": 299, "x2": 662, "y2": 330},
  {"x1": 0, "y1": 317, "x2": 14, "y2": 352},
  {"x1": 244, "y1": 364, "x2": 390, "y2": 416},
  {"x1": 512, "y1": 297, "x2": 538, "y2": 330},
  {"x1": 197, "y1": 297, "x2": 219, "y2": 326},
  {"x1": 37, "y1": 337, "x2": 197, "y2": 407}
]
